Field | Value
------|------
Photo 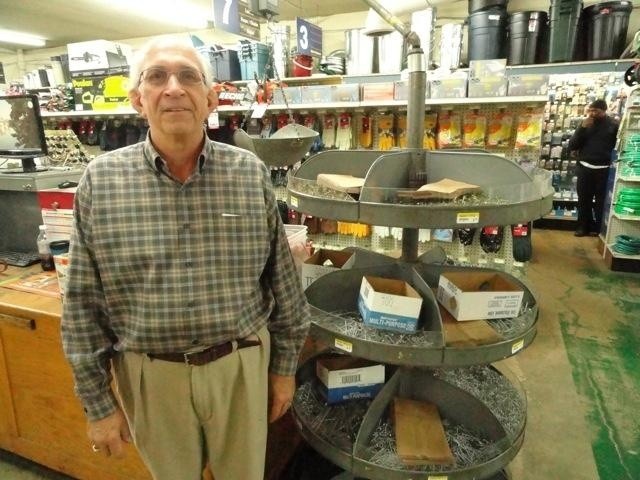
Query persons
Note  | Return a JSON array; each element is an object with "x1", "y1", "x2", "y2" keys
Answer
[
  {"x1": 58, "y1": 34, "x2": 313, "y2": 480},
  {"x1": 569, "y1": 99, "x2": 618, "y2": 236}
]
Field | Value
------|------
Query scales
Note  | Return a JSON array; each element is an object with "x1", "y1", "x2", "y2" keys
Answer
[{"x1": 235, "y1": 0, "x2": 321, "y2": 167}]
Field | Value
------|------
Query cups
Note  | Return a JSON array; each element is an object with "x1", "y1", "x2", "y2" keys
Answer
[{"x1": 49, "y1": 240, "x2": 69, "y2": 257}]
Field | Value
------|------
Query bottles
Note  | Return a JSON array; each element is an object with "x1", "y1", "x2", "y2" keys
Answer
[{"x1": 35, "y1": 224, "x2": 56, "y2": 273}]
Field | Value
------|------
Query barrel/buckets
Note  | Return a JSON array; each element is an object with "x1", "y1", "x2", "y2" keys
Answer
[
  {"x1": 290, "y1": 55, "x2": 313, "y2": 76},
  {"x1": 466, "y1": 0, "x2": 634, "y2": 64},
  {"x1": 285, "y1": 224, "x2": 308, "y2": 285}
]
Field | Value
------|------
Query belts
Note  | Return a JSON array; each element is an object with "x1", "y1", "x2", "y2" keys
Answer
[{"x1": 134, "y1": 335, "x2": 263, "y2": 367}]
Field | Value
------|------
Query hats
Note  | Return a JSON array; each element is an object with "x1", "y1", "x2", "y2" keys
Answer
[{"x1": 588, "y1": 100, "x2": 608, "y2": 111}]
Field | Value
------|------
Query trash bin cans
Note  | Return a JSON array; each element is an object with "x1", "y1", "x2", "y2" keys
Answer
[{"x1": 468, "y1": 0, "x2": 633, "y2": 67}]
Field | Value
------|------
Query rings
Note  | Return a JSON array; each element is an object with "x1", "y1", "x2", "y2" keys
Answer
[{"x1": 91, "y1": 445, "x2": 100, "y2": 452}]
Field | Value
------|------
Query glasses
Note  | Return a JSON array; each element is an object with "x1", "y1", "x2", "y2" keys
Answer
[{"x1": 137, "y1": 67, "x2": 209, "y2": 88}]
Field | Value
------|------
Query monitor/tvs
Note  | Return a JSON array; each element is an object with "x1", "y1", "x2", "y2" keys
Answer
[{"x1": 0, "y1": 94, "x2": 49, "y2": 174}]
